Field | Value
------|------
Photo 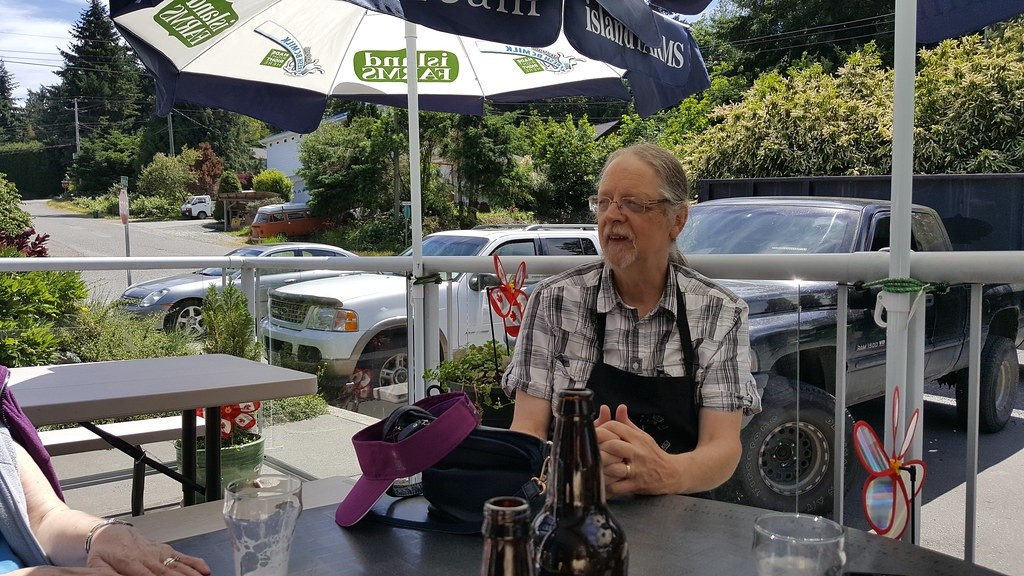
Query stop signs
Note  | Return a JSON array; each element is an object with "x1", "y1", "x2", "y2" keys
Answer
[{"x1": 118, "y1": 190, "x2": 129, "y2": 225}]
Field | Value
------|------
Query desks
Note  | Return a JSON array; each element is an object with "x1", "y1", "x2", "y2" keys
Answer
[
  {"x1": 164, "y1": 501, "x2": 1010, "y2": 576},
  {"x1": 8, "y1": 354, "x2": 317, "y2": 506}
]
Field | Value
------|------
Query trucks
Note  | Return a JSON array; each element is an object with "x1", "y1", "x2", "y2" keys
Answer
[{"x1": 180, "y1": 195, "x2": 217, "y2": 219}]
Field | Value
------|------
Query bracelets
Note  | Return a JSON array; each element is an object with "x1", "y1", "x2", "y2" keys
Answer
[{"x1": 86, "y1": 518, "x2": 134, "y2": 552}]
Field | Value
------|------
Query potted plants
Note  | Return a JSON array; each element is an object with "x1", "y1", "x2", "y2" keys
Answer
[
  {"x1": 421, "y1": 338, "x2": 514, "y2": 430},
  {"x1": 172, "y1": 274, "x2": 267, "y2": 505}
]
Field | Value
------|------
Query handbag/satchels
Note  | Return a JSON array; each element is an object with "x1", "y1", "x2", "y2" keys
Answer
[{"x1": 380, "y1": 406, "x2": 551, "y2": 532}]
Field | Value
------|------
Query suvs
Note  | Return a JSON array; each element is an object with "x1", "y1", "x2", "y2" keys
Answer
[{"x1": 259, "y1": 223, "x2": 604, "y2": 400}]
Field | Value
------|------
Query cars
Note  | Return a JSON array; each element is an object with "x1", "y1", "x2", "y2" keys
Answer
[
  {"x1": 251, "y1": 202, "x2": 329, "y2": 242},
  {"x1": 117, "y1": 242, "x2": 385, "y2": 342}
]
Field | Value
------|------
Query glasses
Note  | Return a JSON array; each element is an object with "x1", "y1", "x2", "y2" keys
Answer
[{"x1": 588, "y1": 196, "x2": 669, "y2": 214}]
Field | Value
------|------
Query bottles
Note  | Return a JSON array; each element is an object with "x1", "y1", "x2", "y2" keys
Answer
[
  {"x1": 534, "y1": 389, "x2": 629, "y2": 576},
  {"x1": 480, "y1": 496, "x2": 534, "y2": 576}
]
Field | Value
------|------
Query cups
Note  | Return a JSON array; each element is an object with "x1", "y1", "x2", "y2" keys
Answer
[
  {"x1": 222, "y1": 474, "x2": 302, "y2": 576},
  {"x1": 753, "y1": 512, "x2": 847, "y2": 576}
]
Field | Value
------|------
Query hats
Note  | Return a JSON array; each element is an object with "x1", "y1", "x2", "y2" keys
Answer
[{"x1": 334, "y1": 391, "x2": 478, "y2": 527}]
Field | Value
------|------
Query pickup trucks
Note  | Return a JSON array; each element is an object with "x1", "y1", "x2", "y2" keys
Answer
[{"x1": 505, "y1": 195, "x2": 1024, "y2": 522}]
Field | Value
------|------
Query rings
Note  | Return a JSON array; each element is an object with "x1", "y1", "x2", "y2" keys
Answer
[
  {"x1": 623, "y1": 462, "x2": 632, "y2": 479},
  {"x1": 163, "y1": 557, "x2": 177, "y2": 567}
]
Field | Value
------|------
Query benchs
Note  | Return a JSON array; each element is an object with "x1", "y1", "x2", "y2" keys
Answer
[{"x1": 37, "y1": 417, "x2": 357, "y2": 542}]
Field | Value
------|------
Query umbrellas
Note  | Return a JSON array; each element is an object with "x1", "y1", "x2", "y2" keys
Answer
[{"x1": 108, "y1": 0, "x2": 711, "y2": 402}]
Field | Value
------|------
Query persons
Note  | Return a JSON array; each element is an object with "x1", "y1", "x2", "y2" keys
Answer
[
  {"x1": 0, "y1": 364, "x2": 212, "y2": 576},
  {"x1": 502, "y1": 142, "x2": 765, "y2": 500}
]
